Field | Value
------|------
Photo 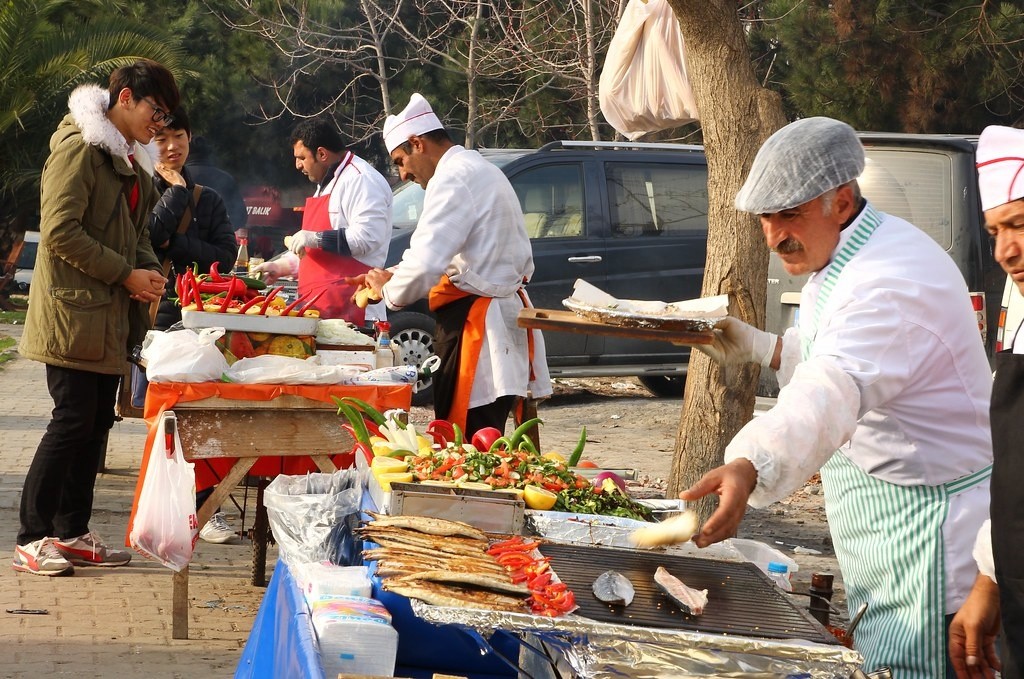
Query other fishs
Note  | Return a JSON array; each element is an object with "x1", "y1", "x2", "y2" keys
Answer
[{"x1": 351, "y1": 509, "x2": 533, "y2": 614}]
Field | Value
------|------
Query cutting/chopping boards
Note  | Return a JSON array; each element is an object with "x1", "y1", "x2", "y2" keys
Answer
[{"x1": 518, "y1": 307, "x2": 715, "y2": 345}]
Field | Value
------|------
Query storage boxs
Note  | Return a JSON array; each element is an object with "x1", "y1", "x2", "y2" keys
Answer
[{"x1": 728, "y1": 537, "x2": 799, "y2": 579}]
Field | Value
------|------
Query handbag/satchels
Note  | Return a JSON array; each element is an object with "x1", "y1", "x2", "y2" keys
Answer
[
  {"x1": 222, "y1": 355, "x2": 360, "y2": 384},
  {"x1": 130, "y1": 410, "x2": 199, "y2": 572},
  {"x1": 139, "y1": 326, "x2": 231, "y2": 383},
  {"x1": 598, "y1": 0, "x2": 698, "y2": 141},
  {"x1": 343, "y1": 355, "x2": 441, "y2": 394},
  {"x1": 323, "y1": 509, "x2": 363, "y2": 566},
  {"x1": 130, "y1": 352, "x2": 149, "y2": 410}
]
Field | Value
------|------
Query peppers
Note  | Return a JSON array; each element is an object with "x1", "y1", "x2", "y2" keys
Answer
[
  {"x1": 296, "y1": 288, "x2": 328, "y2": 316},
  {"x1": 484, "y1": 535, "x2": 574, "y2": 617},
  {"x1": 426, "y1": 419, "x2": 588, "y2": 466},
  {"x1": 281, "y1": 289, "x2": 313, "y2": 316},
  {"x1": 329, "y1": 395, "x2": 424, "y2": 466},
  {"x1": 167, "y1": 261, "x2": 284, "y2": 315}
]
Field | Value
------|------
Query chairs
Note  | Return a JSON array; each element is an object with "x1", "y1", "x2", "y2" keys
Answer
[{"x1": 521, "y1": 210, "x2": 584, "y2": 239}]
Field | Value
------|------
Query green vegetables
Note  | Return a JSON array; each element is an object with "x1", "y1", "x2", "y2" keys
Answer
[{"x1": 407, "y1": 452, "x2": 652, "y2": 522}]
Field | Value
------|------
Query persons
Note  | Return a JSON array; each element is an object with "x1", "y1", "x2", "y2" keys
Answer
[
  {"x1": 144, "y1": 104, "x2": 237, "y2": 544},
  {"x1": 948, "y1": 123, "x2": 1024, "y2": 678},
  {"x1": 668, "y1": 114, "x2": 997, "y2": 679},
  {"x1": 345, "y1": 93, "x2": 553, "y2": 446},
  {"x1": 251, "y1": 118, "x2": 393, "y2": 330},
  {"x1": 12, "y1": 58, "x2": 180, "y2": 576}
]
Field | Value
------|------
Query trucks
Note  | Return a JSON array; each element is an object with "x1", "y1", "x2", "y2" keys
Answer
[{"x1": 239, "y1": 153, "x2": 316, "y2": 260}]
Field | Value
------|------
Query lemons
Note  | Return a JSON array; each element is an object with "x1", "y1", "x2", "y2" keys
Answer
[
  {"x1": 371, "y1": 434, "x2": 568, "y2": 510},
  {"x1": 182, "y1": 297, "x2": 321, "y2": 318}
]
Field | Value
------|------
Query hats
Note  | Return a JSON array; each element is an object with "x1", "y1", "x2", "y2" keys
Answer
[
  {"x1": 976, "y1": 124, "x2": 1024, "y2": 212},
  {"x1": 383, "y1": 92, "x2": 444, "y2": 156},
  {"x1": 733, "y1": 115, "x2": 865, "y2": 213}
]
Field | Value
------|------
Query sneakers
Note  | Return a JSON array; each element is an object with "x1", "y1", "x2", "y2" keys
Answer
[
  {"x1": 12, "y1": 535, "x2": 75, "y2": 576},
  {"x1": 199, "y1": 511, "x2": 239, "y2": 543},
  {"x1": 53, "y1": 531, "x2": 132, "y2": 565}
]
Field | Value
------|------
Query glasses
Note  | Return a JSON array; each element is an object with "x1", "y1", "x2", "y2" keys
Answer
[{"x1": 139, "y1": 95, "x2": 172, "y2": 126}]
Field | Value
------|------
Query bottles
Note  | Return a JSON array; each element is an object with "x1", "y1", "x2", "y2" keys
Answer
[
  {"x1": 765, "y1": 562, "x2": 792, "y2": 597},
  {"x1": 377, "y1": 330, "x2": 390, "y2": 349},
  {"x1": 234, "y1": 240, "x2": 248, "y2": 275}
]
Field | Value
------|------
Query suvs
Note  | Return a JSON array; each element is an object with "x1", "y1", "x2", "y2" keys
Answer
[{"x1": 385, "y1": 141, "x2": 709, "y2": 406}]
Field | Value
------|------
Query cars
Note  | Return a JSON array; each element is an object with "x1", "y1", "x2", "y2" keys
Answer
[{"x1": 14, "y1": 231, "x2": 41, "y2": 291}]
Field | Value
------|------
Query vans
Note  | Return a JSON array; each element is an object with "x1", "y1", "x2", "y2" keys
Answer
[{"x1": 853, "y1": 130, "x2": 1007, "y2": 373}]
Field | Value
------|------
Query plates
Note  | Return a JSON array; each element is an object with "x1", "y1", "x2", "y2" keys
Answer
[{"x1": 562, "y1": 298, "x2": 726, "y2": 332}]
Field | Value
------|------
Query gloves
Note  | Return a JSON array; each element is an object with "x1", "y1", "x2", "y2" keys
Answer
[
  {"x1": 250, "y1": 260, "x2": 282, "y2": 284},
  {"x1": 288, "y1": 229, "x2": 324, "y2": 254}
]
